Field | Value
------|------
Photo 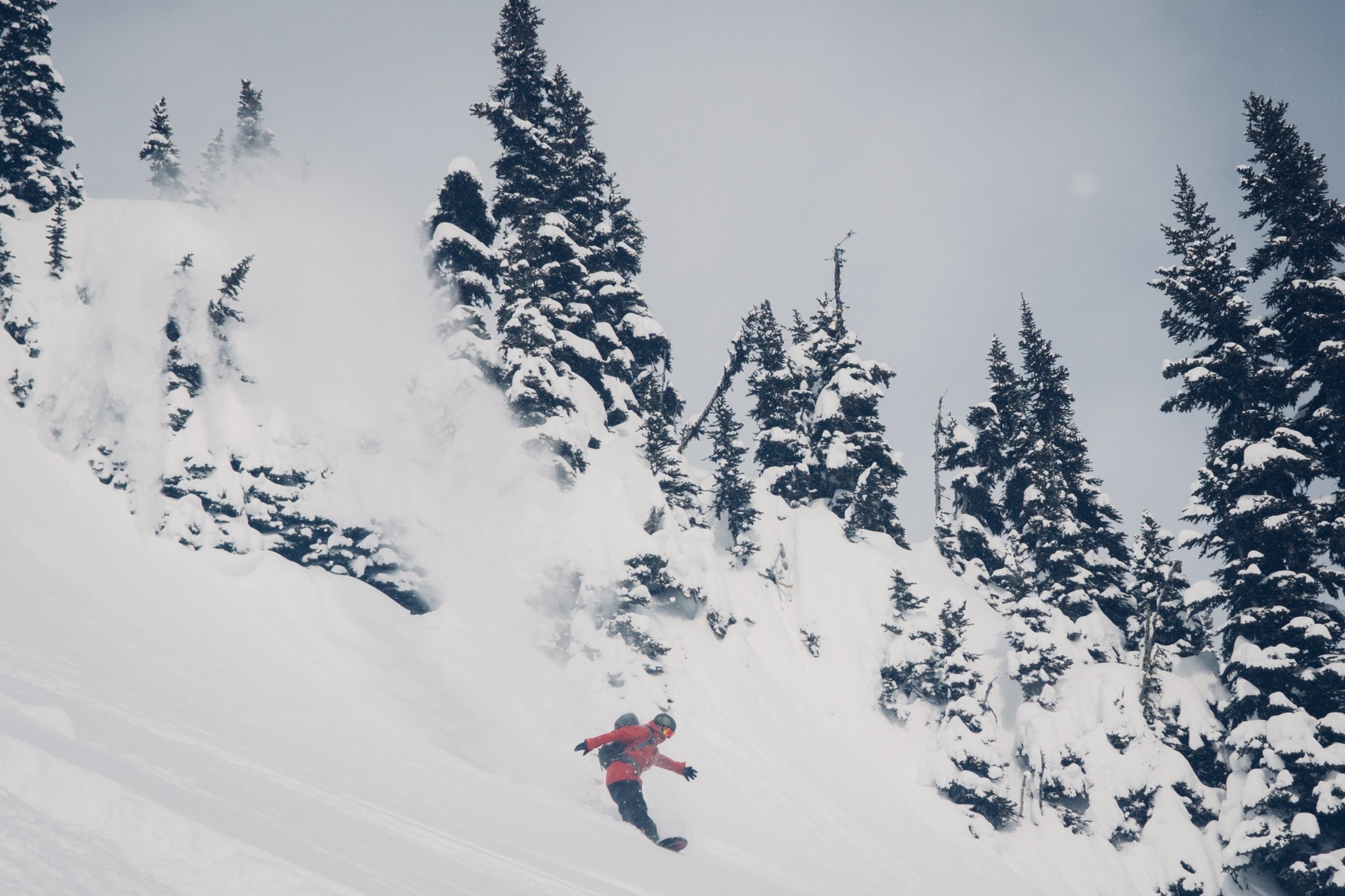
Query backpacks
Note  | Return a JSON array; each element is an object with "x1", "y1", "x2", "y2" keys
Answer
[{"x1": 598, "y1": 713, "x2": 653, "y2": 770}]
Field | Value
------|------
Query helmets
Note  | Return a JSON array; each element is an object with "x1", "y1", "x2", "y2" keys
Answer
[{"x1": 653, "y1": 713, "x2": 676, "y2": 732}]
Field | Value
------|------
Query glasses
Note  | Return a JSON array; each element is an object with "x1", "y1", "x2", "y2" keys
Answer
[{"x1": 663, "y1": 728, "x2": 674, "y2": 739}]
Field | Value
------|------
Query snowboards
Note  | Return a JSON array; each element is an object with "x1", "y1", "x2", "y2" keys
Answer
[{"x1": 652, "y1": 837, "x2": 688, "y2": 852}]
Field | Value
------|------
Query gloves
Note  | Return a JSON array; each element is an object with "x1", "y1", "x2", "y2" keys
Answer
[
  {"x1": 683, "y1": 766, "x2": 698, "y2": 781},
  {"x1": 574, "y1": 741, "x2": 588, "y2": 756}
]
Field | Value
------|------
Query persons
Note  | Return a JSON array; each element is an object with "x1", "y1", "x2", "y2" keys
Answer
[{"x1": 574, "y1": 714, "x2": 698, "y2": 852}]
